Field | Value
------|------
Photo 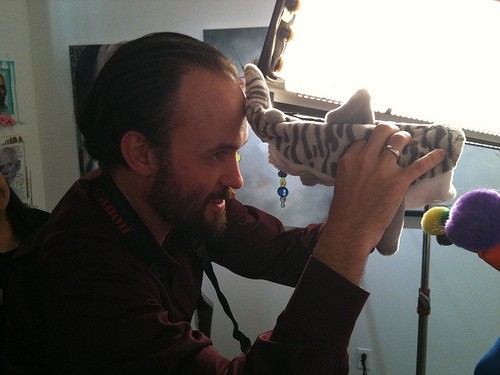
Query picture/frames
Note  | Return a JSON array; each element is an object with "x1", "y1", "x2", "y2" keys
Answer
[
  {"x1": 404, "y1": 204, "x2": 430, "y2": 217},
  {"x1": 0, "y1": 60, "x2": 19, "y2": 127},
  {"x1": 0, "y1": 135, "x2": 33, "y2": 205}
]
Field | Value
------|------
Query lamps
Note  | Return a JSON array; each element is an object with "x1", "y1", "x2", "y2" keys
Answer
[{"x1": 251, "y1": 0, "x2": 433, "y2": 375}]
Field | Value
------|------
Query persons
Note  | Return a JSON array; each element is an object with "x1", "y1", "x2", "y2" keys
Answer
[
  {"x1": 0, "y1": 31, "x2": 447, "y2": 375},
  {"x1": 0, "y1": 168, "x2": 53, "y2": 309},
  {"x1": 0, "y1": 73, "x2": 9, "y2": 113},
  {"x1": 0, "y1": 146, "x2": 21, "y2": 186}
]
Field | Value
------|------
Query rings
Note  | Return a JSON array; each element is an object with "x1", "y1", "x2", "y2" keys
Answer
[{"x1": 383, "y1": 144, "x2": 401, "y2": 158}]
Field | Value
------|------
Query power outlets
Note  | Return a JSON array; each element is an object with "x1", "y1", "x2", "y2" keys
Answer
[{"x1": 356, "y1": 347, "x2": 371, "y2": 370}]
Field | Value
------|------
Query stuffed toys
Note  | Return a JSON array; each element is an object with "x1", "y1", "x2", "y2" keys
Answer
[
  {"x1": 241, "y1": 63, "x2": 467, "y2": 258},
  {"x1": 421, "y1": 187, "x2": 500, "y2": 375}
]
